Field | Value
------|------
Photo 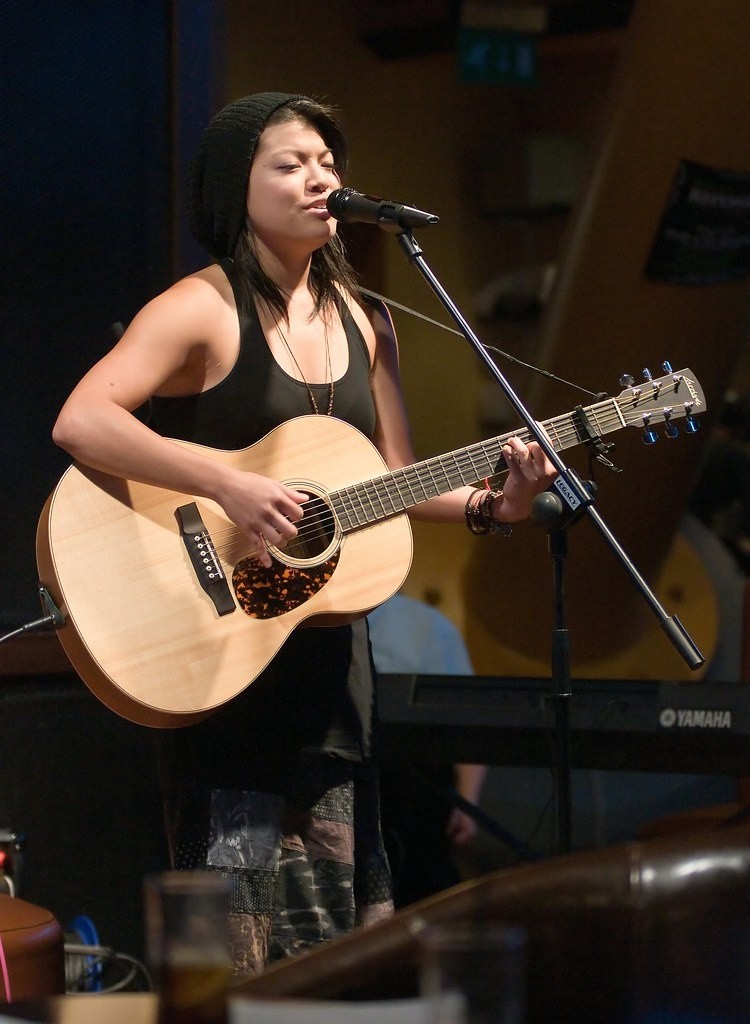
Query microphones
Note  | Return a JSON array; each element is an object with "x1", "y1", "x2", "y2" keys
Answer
[{"x1": 326, "y1": 186, "x2": 440, "y2": 225}]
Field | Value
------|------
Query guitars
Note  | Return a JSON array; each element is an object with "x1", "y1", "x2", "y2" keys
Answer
[{"x1": 35, "y1": 358, "x2": 708, "y2": 731}]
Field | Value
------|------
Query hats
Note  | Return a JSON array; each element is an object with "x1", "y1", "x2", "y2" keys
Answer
[{"x1": 188, "y1": 91, "x2": 308, "y2": 258}]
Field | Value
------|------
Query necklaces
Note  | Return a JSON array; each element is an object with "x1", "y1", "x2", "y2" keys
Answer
[{"x1": 258, "y1": 291, "x2": 334, "y2": 418}]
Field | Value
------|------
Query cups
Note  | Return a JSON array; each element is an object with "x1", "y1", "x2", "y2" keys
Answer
[{"x1": 152, "y1": 873, "x2": 234, "y2": 1024}]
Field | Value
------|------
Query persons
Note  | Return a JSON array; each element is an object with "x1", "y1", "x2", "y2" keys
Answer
[
  {"x1": 53, "y1": 91, "x2": 563, "y2": 980},
  {"x1": 362, "y1": 591, "x2": 487, "y2": 915}
]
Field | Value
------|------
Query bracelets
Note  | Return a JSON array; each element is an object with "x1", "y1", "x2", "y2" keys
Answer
[{"x1": 465, "y1": 477, "x2": 514, "y2": 538}]
